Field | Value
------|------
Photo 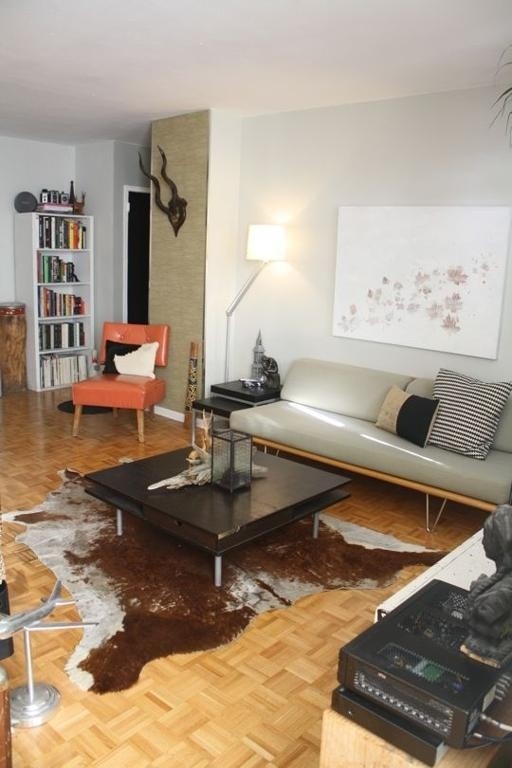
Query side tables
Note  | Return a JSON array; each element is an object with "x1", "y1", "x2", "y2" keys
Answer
[{"x1": 210, "y1": 378, "x2": 284, "y2": 447}]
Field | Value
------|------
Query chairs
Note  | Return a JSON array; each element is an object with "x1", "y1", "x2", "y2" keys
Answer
[{"x1": 71, "y1": 322, "x2": 169, "y2": 441}]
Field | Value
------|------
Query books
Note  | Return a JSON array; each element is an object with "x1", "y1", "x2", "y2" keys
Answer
[{"x1": 37, "y1": 215, "x2": 90, "y2": 390}]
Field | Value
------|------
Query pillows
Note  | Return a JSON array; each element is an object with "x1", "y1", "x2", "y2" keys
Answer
[
  {"x1": 375, "y1": 368, "x2": 511, "y2": 462},
  {"x1": 101, "y1": 339, "x2": 161, "y2": 380}
]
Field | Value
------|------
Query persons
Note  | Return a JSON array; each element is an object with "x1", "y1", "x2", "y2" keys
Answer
[{"x1": 462, "y1": 503, "x2": 512, "y2": 661}]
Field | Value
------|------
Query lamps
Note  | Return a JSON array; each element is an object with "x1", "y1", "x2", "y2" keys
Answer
[{"x1": 223, "y1": 221, "x2": 290, "y2": 379}]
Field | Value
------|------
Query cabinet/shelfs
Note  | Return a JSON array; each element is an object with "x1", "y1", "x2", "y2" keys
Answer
[{"x1": 14, "y1": 213, "x2": 96, "y2": 393}]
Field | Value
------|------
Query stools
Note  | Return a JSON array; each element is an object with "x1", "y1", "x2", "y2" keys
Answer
[{"x1": 191, "y1": 396, "x2": 253, "y2": 446}]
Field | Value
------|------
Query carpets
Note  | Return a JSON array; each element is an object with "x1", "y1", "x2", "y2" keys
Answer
[{"x1": 0, "y1": 456, "x2": 448, "y2": 694}]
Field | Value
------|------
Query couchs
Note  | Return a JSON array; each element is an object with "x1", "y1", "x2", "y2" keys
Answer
[{"x1": 229, "y1": 358, "x2": 511, "y2": 534}]
